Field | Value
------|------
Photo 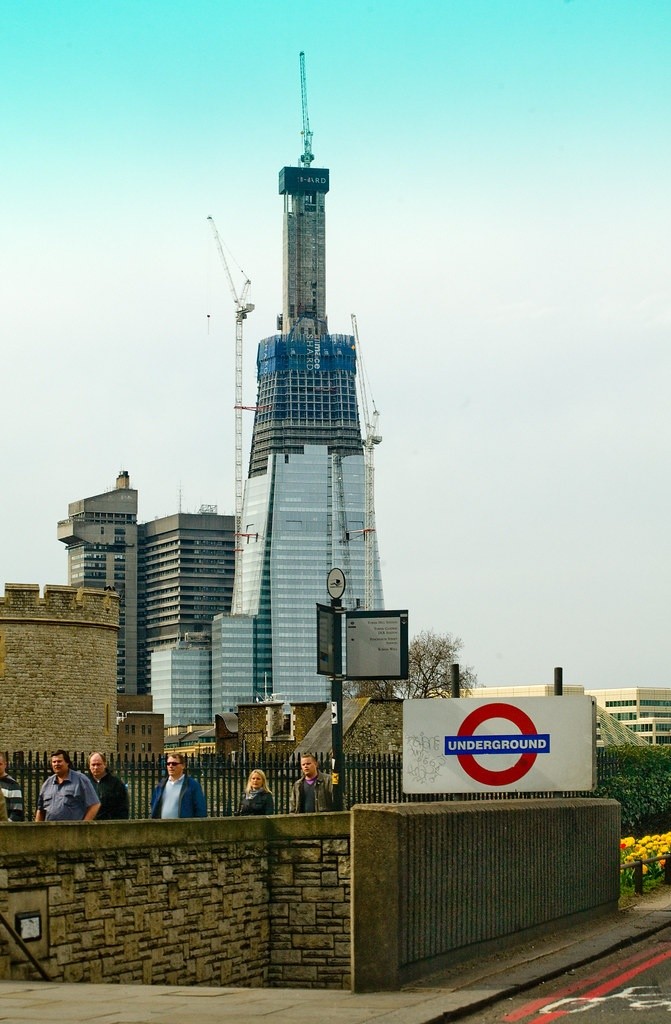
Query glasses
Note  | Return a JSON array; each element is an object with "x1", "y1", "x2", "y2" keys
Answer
[{"x1": 166, "y1": 762, "x2": 182, "y2": 766}]
